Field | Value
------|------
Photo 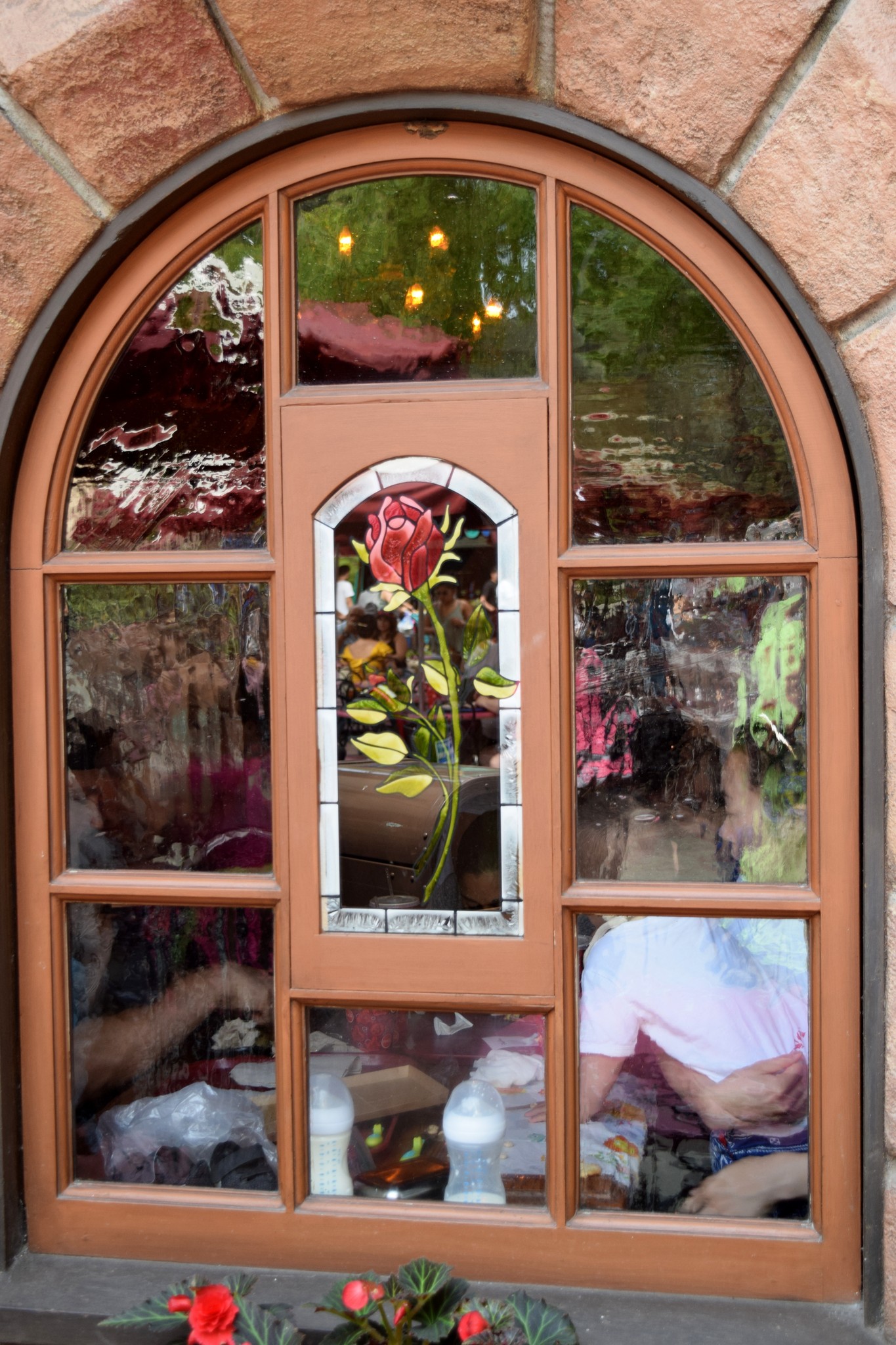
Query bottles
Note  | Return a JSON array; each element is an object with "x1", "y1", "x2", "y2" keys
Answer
[
  {"x1": 309, "y1": 1071, "x2": 355, "y2": 1196},
  {"x1": 441, "y1": 1078, "x2": 507, "y2": 1205}
]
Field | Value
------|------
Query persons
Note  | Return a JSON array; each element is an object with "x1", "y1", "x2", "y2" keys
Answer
[
  {"x1": 61, "y1": 704, "x2": 813, "y2": 1219},
  {"x1": 196, "y1": 496, "x2": 807, "y2": 774}
]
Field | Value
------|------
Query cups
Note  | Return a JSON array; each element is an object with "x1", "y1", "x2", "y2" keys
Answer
[{"x1": 369, "y1": 895, "x2": 420, "y2": 909}]
